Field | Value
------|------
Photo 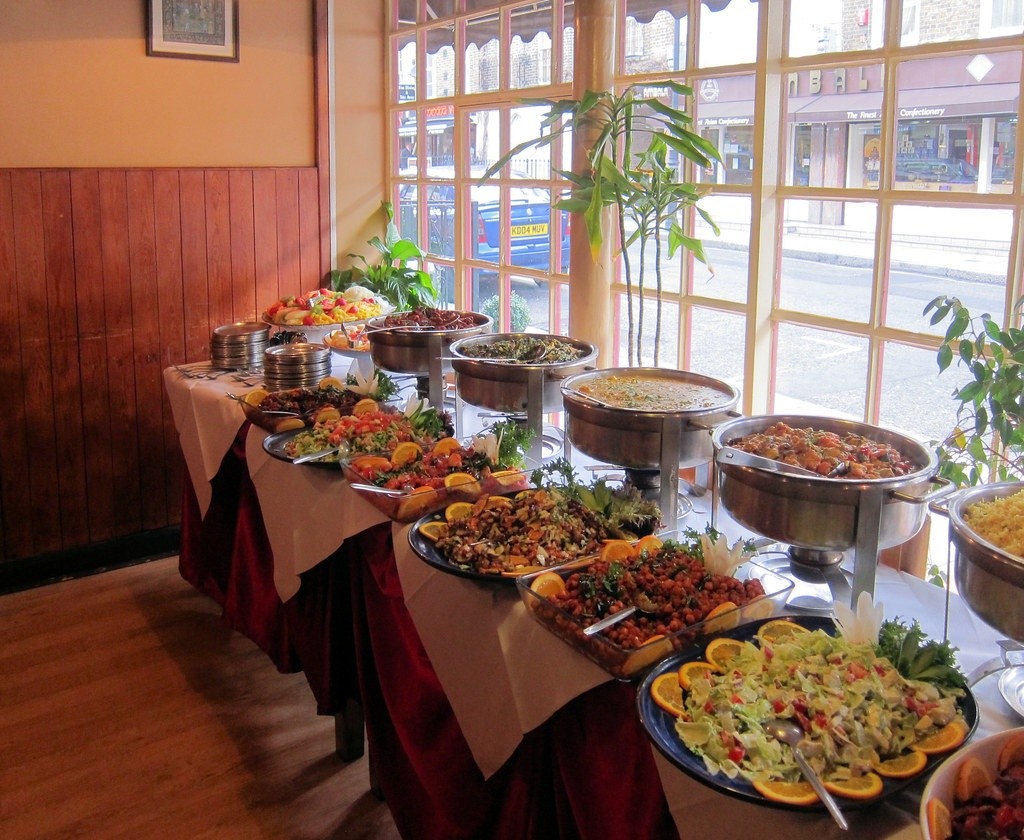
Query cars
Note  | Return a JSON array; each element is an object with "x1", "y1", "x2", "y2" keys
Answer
[
  {"x1": 726, "y1": 152, "x2": 754, "y2": 184},
  {"x1": 869, "y1": 158, "x2": 1006, "y2": 185},
  {"x1": 399, "y1": 162, "x2": 571, "y2": 304}
]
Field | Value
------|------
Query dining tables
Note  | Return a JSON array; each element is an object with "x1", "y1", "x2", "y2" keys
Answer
[{"x1": 164, "y1": 360, "x2": 1024, "y2": 840}]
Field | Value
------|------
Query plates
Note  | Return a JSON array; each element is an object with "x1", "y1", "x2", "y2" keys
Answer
[
  {"x1": 514, "y1": 529, "x2": 796, "y2": 680},
  {"x1": 262, "y1": 343, "x2": 332, "y2": 392},
  {"x1": 635, "y1": 615, "x2": 981, "y2": 816},
  {"x1": 210, "y1": 322, "x2": 272, "y2": 369},
  {"x1": 261, "y1": 425, "x2": 455, "y2": 471},
  {"x1": 339, "y1": 434, "x2": 545, "y2": 523},
  {"x1": 239, "y1": 383, "x2": 404, "y2": 434},
  {"x1": 406, "y1": 486, "x2": 654, "y2": 586}
]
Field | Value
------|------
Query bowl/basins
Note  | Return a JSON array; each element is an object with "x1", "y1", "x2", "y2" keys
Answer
[{"x1": 919, "y1": 727, "x2": 1024, "y2": 840}]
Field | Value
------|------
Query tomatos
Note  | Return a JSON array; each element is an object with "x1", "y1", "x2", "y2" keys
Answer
[{"x1": 270, "y1": 288, "x2": 376, "y2": 326}]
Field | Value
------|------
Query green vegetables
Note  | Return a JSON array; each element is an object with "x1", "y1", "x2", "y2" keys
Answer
[
  {"x1": 290, "y1": 397, "x2": 664, "y2": 539},
  {"x1": 875, "y1": 615, "x2": 968, "y2": 699},
  {"x1": 344, "y1": 369, "x2": 398, "y2": 401},
  {"x1": 577, "y1": 523, "x2": 759, "y2": 616}
]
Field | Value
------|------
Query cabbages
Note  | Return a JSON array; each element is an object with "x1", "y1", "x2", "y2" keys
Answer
[{"x1": 673, "y1": 629, "x2": 962, "y2": 785}]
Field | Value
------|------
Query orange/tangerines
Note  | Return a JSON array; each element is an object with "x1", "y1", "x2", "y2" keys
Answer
[
  {"x1": 483, "y1": 495, "x2": 511, "y2": 510},
  {"x1": 490, "y1": 470, "x2": 523, "y2": 485},
  {"x1": 753, "y1": 723, "x2": 964, "y2": 805},
  {"x1": 929, "y1": 798, "x2": 953, "y2": 840},
  {"x1": 243, "y1": 377, "x2": 379, "y2": 432},
  {"x1": 502, "y1": 535, "x2": 664, "y2": 601},
  {"x1": 621, "y1": 594, "x2": 813, "y2": 718},
  {"x1": 445, "y1": 502, "x2": 474, "y2": 522},
  {"x1": 390, "y1": 441, "x2": 423, "y2": 466},
  {"x1": 433, "y1": 437, "x2": 463, "y2": 452},
  {"x1": 419, "y1": 521, "x2": 447, "y2": 541},
  {"x1": 514, "y1": 490, "x2": 530, "y2": 498},
  {"x1": 998, "y1": 732, "x2": 1024, "y2": 770},
  {"x1": 444, "y1": 472, "x2": 481, "y2": 491},
  {"x1": 397, "y1": 485, "x2": 437, "y2": 518},
  {"x1": 958, "y1": 758, "x2": 991, "y2": 800}
]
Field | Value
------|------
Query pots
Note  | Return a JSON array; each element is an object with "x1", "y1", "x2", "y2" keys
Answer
[
  {"x1": 559, "y1": 366, "x2": 741, "y2": 471},
  {"x1": 366, "y1": 309, "x2": 495, "y2": 375},
  {"x1": 708, "y1": 414, "x2": 958, "y2": 552},
  {"x1": 448, "y1": 332, "x2": 600, "y2": 414},
  {"x1": 927, "y1": 480, "x2": 1024, "y2": 647}
]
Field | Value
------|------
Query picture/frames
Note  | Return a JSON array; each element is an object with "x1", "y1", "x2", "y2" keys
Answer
[{"x1": 145, "y1": 0, "x2": 241, "y2": 63}]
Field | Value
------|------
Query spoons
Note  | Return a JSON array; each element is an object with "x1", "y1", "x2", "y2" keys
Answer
[
  {"x1": 293, "y1": 436, "x2": 351, "y2": 464},
  {"x1": 763, "y1": 719, "x2": 848, "y2": 832}
]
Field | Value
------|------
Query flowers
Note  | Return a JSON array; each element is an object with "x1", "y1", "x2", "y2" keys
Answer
[
  {"x1": 405, "y1": 392, "x2": 435, "y2": 427},
  {"x1": 700, "y1": 533, "x2": 751, "y2": 575},
  {"x1": 347, "y1": 369, "x2": 379, "y2": 398},
  {"x1": 830, "y1": 591, "x2": 885, "y2": 646},
  {"x1": 470, "y1": 428, "x2": 504, "y2": 465}
]
